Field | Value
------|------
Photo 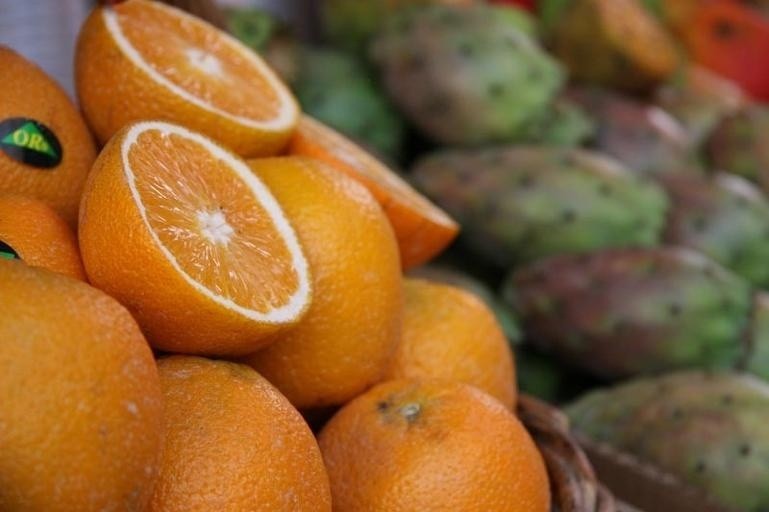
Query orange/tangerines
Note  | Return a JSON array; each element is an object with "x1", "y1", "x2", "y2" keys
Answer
[{"x1": 0, "y1": 1, "x2": 551, "y2": 511}]
